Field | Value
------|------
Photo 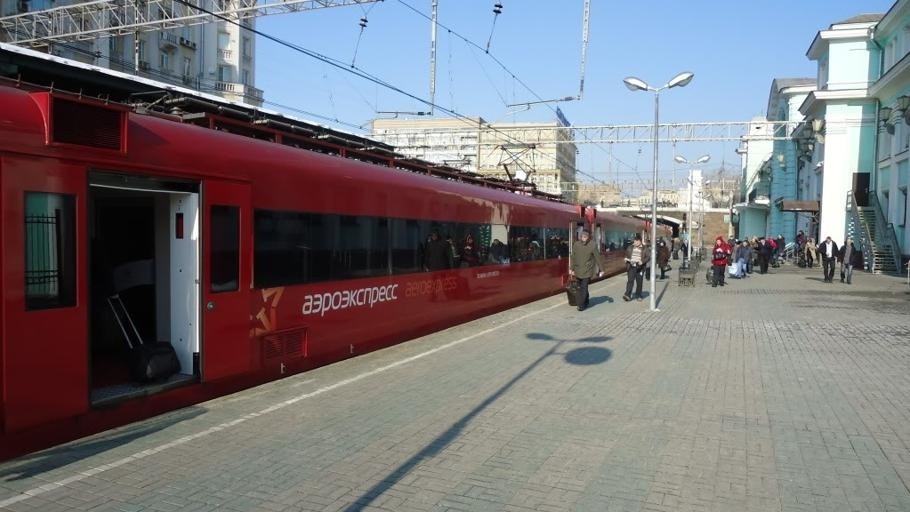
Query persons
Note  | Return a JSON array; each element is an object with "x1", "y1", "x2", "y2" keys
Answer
[
  {"x1": 816, "y1": 235, "x2": 839, "y2": 283},
  {"x1": 838, "y1": 237, "x2": 858, "y2": 285},
  {"x1": 711, "y1": 236, "x2": 733, "y2": 287},
  {"x1": 805, "y1": 238, "x2": 817, "y2": 269},
  {"x1": 727, "y1": 233, "x2": 786, "y2": 277},
  {"x1": 644, "y1": 239, "x2": 652, "y2": 280},
  {"x1": 794, "y1": 229, "x2": 805, "y2": 253},
  {"x1": 419, "y1": 229, "x2": 569, "y2": 272},
  {"x1": 606, "y1": 240, "x2": 617, "y2": 252},
  {"x1": 569, "y1": 229, "x2": 606, "y2": 312},
  {"x1": 656, "y1": 239, "x2": 670, "y2": 280},
  {"x1": 671, "y1": 236, "x2": 681, "y2": 260},
  {"x1": 623, "y1": 234, "x2": 652, "y2": 303},
  {"x1": 681, "y1": 239, "x2": 694, "y2": 265}
]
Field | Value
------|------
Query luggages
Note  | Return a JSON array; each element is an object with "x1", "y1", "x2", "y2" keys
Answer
[
  {"x1": 123, "y1": 339, "x2": 182, "y2": 388},
  {"x1": 565, "y1": 269, "x2": 590, "y2": 307},
  {"x1": 706, "y1": 266, "x2": 715, "y2": 284}
]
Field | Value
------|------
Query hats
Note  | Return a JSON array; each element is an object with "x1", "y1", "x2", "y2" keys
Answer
[{"x1": 716, "y1": 235, "x2": 723, "y2": 241}]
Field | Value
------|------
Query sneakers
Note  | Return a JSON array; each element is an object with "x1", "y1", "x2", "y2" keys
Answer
[
  {"x1": 623, "y1": 295, "x2": 643, "y2": 302},
  {"x1": 825, "y1": 278, "x2": 851, "y2": 284}
]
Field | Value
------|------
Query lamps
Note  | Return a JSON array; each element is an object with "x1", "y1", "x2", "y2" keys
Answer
[{"x1": 880, "y1": 94, "x2": 910, "y2": 127}]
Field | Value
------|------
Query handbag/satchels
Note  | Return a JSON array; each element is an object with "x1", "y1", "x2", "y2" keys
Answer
[
  {"x1": 712, "y1": 251, "x2": 726, "y2": 260},
  {"x1": 662, "y1": 263, "x2": 672, "y2": 271}
]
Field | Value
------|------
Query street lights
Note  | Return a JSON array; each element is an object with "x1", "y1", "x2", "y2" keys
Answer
[
  {"x1": 623, "y1": 71, "x2": 694, "y2": 310},
  {"x1": 674, "y1": 154, "x2": 712, "y2": 261}
]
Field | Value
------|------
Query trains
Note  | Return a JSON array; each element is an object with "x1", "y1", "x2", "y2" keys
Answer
[{"x1": 0, "y1": 83, "x2": 672, "y2": 465}]
